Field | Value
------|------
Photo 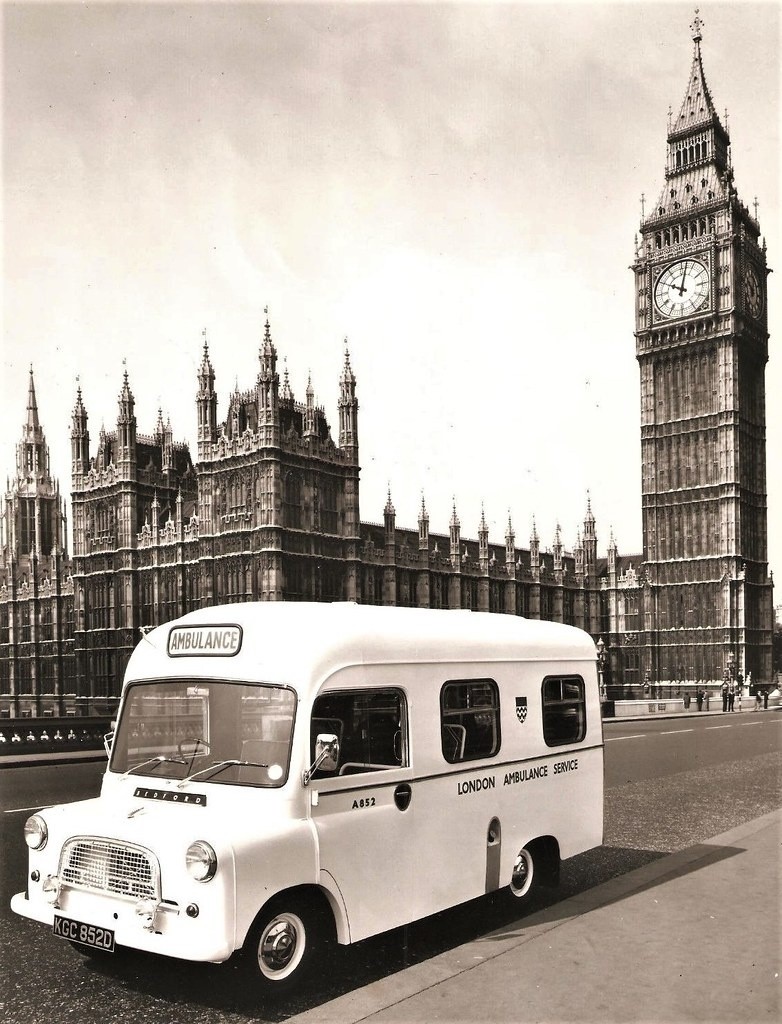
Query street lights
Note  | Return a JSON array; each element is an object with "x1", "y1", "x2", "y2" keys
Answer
[{"x1": 596, "y1": 638, "x2": 607, "y2": 717}]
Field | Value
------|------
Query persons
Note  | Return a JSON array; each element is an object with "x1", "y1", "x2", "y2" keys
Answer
[
  {"x1": 722, "y1": 690, "x2": 730, "y2": 712},
  {"x1": 756, "y1": 691, "x2": 762, "y2": 711},
  {"x1": 703, "y1": 690, "x2": 710, "y2": 711},
  {"x1": 728, "y1": 690, "x2": 735, "y2": 712},
  {"x1": 696, "y1": 690, "x2": 704, "y2": 711},
  {"x1": 683, "y1": 691, "x2": 692, "y2": 714},
  {"x1": 764, "y1": 690, "x2": 769, "y2": 709}
]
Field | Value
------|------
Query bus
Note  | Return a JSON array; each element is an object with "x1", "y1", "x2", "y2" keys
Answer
[{"x1": 9, "y1": 600, "x2": 607, "y2": 1001}]
